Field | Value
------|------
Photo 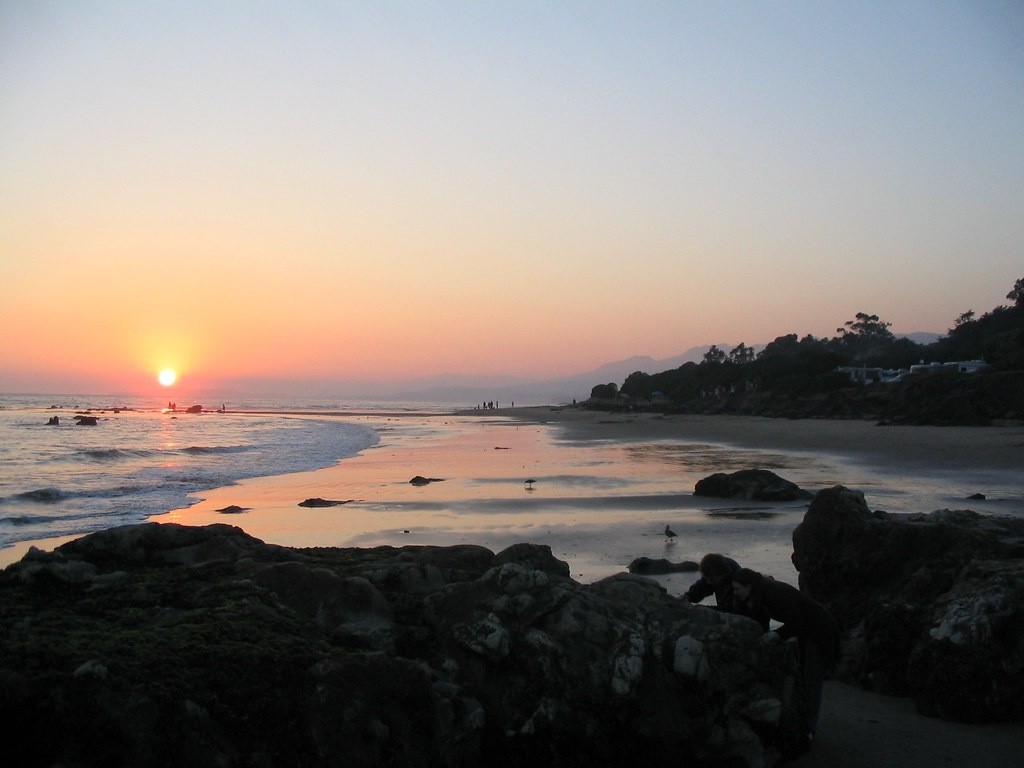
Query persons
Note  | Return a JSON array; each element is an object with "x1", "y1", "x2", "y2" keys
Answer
[
  {"x1": 512, "y1": 401, "x2": 514, "y2": 407},
  {"x1": 221, "y1": 404, "x2": 226, "y2": 413},
  {"x1": 478, "y1": 400, "x2": 498, "y2": 410},
  {"x1": 573, "y1": 398, "x2": 576, "y2": 405}
]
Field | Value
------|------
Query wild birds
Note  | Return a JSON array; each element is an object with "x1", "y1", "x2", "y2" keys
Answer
[
  {"x1": 524, "y1": 479, "x2": 536, "y2": 486},
  {"x1": 664, "y1": 524, "x2": 678, "y2": 542}
]
